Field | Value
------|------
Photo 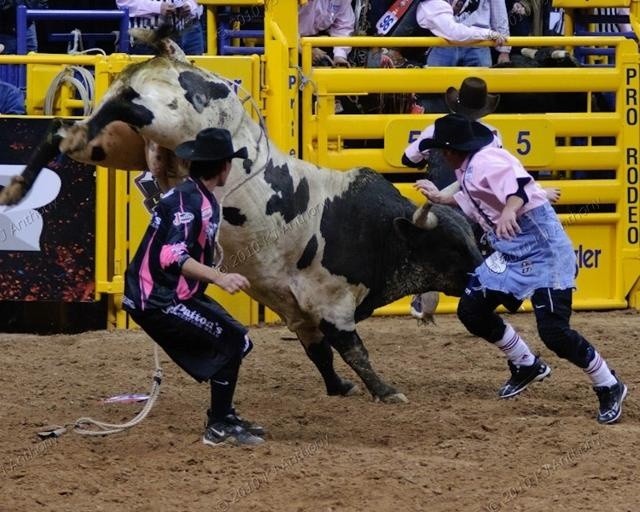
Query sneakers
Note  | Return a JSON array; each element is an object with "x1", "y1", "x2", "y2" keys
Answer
[
  {"x1": 498, "y1": 350, "x2": 551, "y2": 398},
  {"x1": 228, "y1": 409, "x2": 264, "y2": 436},
  {"x1": 592, "y1": 369, "x2": 627, "y2": 424},
  {"x1": 203, "y1": 419, "x2": 264, "y2": 449}
]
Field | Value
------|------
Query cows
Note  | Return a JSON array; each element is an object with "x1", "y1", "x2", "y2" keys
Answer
[{"x1": 0, "y1": 9, "x2": 484, "y2": 404}]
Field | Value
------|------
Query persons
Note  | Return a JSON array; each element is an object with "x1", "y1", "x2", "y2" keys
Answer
[
  {"x1": 367, "y1": 1, "x2": 508, "y2": 68},
  {"x1": 0, "y1": 43, "x2": 27, "y2": 114},
  {"x1": 115, "y1": 0, "x2": 203, "y2": 56},
  {"x1": 401, "y1": 76, "x2": 560, "y2": 321},
  {"x1": 413, "y1": 114, "x2": 628, "y2": 423},
  {"x1": 427, "y1": 0, "x2": 512, "y2": 68},
  {"x1": 297, "y1": 0, "x2": 357, "y2": 68},
  {"x1": 121, "y1": 128, "x2": 265, "y2": 448},
  {"x1": 0, "y1": 1, "x2": 39, "y2": 54}
]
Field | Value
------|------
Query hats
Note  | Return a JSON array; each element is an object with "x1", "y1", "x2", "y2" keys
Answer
[
  {"x1": 174, "y1": 129, "x2": 248, "y2": 162},
  {"x1": 445, "y1": 77, "x2": 500, "y2": 119},
  {"x1": 419, "y1": 114, "x2": 494, "y2": 151}
]
